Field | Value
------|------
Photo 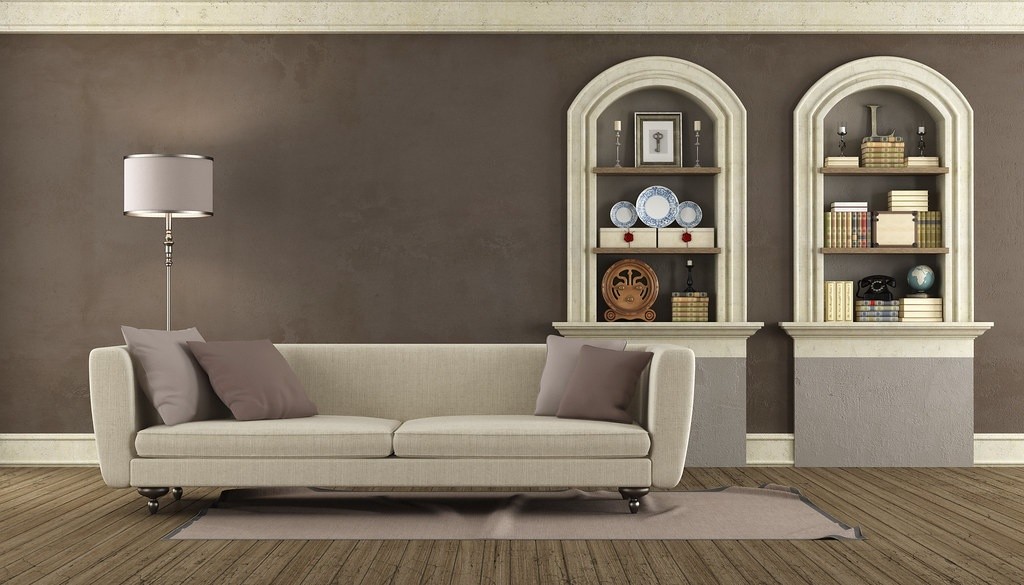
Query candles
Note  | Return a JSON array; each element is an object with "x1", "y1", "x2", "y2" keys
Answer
[
  {"x1": 687, "y1": 260, "x2": 692, "y2": 266},
  {"x1": 614, "y1": 121, "x2": 621, "y2": 132},
  {"x1": 918, "y1": 126, "x2": 925, "y2": 134},
  {"x1": 839, "y1": 126, "x2": 846, "y2": 134},
  {"x1": 693, "y1": 120, "x2": 701, "y2": 131}
]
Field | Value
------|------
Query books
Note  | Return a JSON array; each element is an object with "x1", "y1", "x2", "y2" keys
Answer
[
  {"x1": 670, "y1": 291, "x2": 709, "y2": 322},
  {"x1": 824, "y1": 136, "x2": 943, "y2": 322}
]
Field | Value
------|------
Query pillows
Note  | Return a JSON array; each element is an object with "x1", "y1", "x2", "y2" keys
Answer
[
  {"x1": 556, "y1": 344, "x2": 654, "y2": 424},
  {"x1": 119, "y1": 324, "x2": 230, "y2": 425},
  {"x1": 534, "y1": 334, "x2": 626, "y2": 417},
  {"x1": 186, "y1": 339, "x2": 318, "y2": 422}
]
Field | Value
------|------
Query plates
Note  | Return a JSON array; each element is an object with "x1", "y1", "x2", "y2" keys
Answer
[
  {"x1": 676, "y1": 201, "x2": 702, "y2": 228},
  {"x1": 610, "y1": 201, "x2": 638, "y2": 228},
  {"x1": 636, "y1": 186, "x2": 679, "y2": 228}
]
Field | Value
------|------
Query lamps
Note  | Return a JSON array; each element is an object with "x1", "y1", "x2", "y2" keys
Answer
[{"x1": 123, "y1": 153, "x2": 214, "y2": 331}]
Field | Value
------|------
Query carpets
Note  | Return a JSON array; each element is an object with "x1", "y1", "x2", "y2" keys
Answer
[{"x1": 171, "y1": 479, "x2": 862, "y2": 540}]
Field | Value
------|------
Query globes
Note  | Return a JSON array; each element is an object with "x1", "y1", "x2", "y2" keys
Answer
[{"x1": 905, "y1": 264, "x2": 937, "y2": 298}]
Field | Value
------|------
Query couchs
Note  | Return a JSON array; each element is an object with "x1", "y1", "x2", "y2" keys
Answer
[{"x1": 88, "y1": 343, "x2": 695, "y2": 514}]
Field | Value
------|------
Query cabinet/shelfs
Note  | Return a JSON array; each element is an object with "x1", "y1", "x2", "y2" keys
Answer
[
  {"x1": 591, "y1": 167, "x2": 722, "y2": 254},
  {"x1": 819, "y1": 167, "x2": 950, "y2": 254}
]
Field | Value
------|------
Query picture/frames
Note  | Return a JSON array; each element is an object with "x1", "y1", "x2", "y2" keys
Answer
[{"x1": 634, "y1": 111, "x2": 683, "y2": 168}]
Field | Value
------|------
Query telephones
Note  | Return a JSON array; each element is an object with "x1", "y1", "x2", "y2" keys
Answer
[{"x1": 856, "y1": 274, "x2": 896, "y2": 301}]
